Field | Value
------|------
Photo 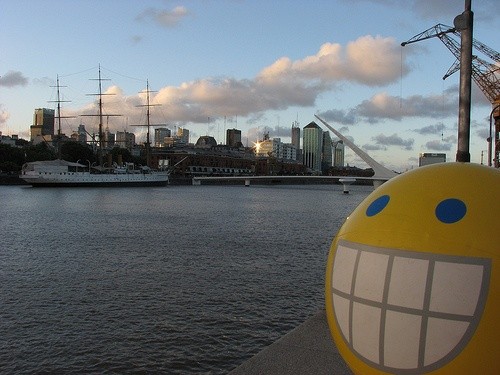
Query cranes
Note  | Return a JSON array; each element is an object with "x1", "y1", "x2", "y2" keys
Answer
[{"x1": 400, "y1": 24, "x2": 500, "y2": 169}]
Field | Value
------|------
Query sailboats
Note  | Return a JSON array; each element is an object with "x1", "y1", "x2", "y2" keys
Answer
[{"x1": 18, "y1": 64, "x2": 175, "y2": 186}]
